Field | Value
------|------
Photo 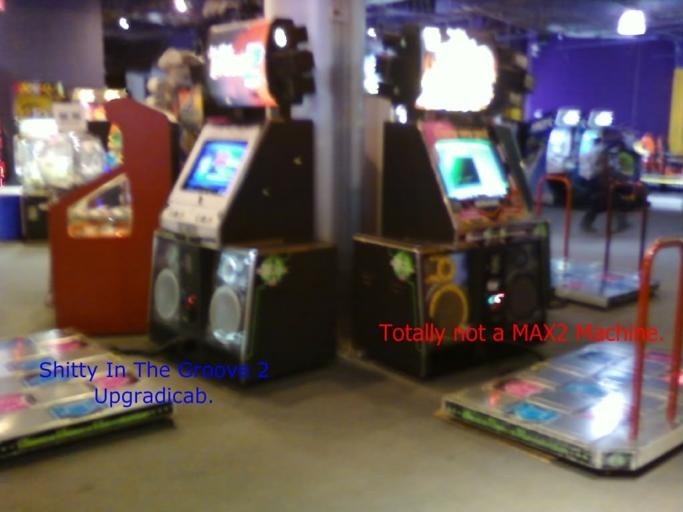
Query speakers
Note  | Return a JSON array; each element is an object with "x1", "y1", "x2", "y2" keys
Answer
[
  {"x1": 204, "y1": 248, "x2": 258, "y2": 354},
  {"x1": 149, "y1": 235, "x2": 202, "y2": 338},
  {"x1": 503, "y1": 242, "x2": 546, "y2": 353},
  {"x1": 419, "y1": 251, "x2": 476, "y2": 379}
]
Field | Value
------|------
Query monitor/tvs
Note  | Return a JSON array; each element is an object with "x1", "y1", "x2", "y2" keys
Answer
[
  {"x1": 435, "y1": 137, "x2": 511, "y2": 202},
  {"x1": 184, "y1": 141, "x2": 247, "y2": 193}
]
